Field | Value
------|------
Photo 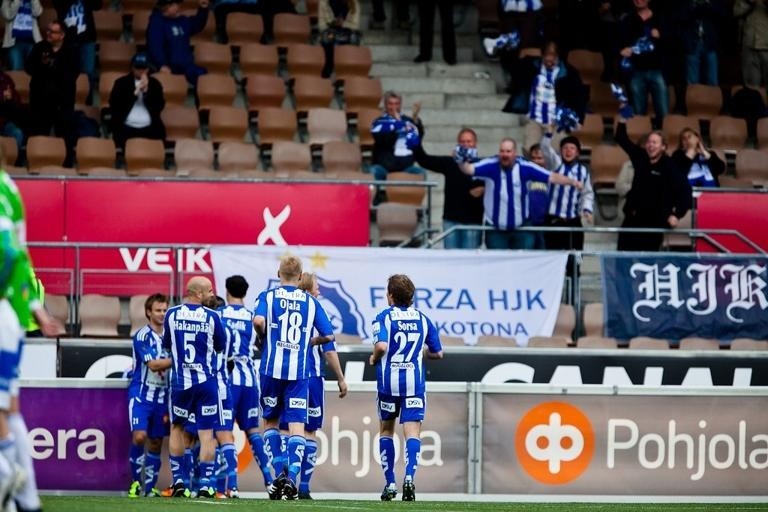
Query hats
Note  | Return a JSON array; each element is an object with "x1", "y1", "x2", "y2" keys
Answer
[
  {"x1": 158, "y1": 0, "x2": 184, "y2": 4},
  {"x1": 225, "y1": 275, "x2": 248, "y2": 298},
  {"x1": 560, "y1": 136, "x2": 579, "y2": 147},
  {"x1": 131, "y1": 53, "x2": 147, "y2": 70}
]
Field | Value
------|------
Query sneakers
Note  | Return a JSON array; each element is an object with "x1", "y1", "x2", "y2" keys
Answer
[
  {"x1": 285, "y1": 479, "x2": 300, "y2": 498},
  {"x1": 268, "y1": 490, "x2": 281, "y2": 500},
  {"x1": 198, "y1": 485, "x2": 211, "y2": 499},
  {"x1": 215, "y1": 490, "x2": 227, "y2": 499},
  {"x1": 381, "y1": 483, "x2": 398, "y2": 501},
  {"x1": 144, "y1": 486, "x2": 160, "y2": 498},
  {"x1": 402, "y1": 480, "x2": 415, "y2": 501},
  {"x1": 160, "y1": 485, "x2": 174, "y2": 498},
  {"x1": 173, "y1": 479, "x2": 186, "y2": 497},
  {"x1": 226, "y1": 486, "x2": 238, "y2": 497},
  {"x1": 266, "y1": 473, "x2": 286, "y2": 499},
  {"x1": 299, "y1": 491, "x2": 313, "y2": 500},
  {"x1": 128, "y1": 481, "x2": 142, "y2": 498},
  {"x1": 0, "y1": 462, "x2": 26, "y2": 512}
]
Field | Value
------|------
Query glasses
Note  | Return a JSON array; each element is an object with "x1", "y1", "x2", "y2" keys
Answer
[{"x1": 46, "y1": 28, "x2": 61, "y2": 33}]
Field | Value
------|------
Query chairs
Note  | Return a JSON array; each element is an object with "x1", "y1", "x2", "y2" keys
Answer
[
  {"x1": 76, "y1": 103, "x2": 100, "y2": 125},
  {"x1": 731, "y1": 83, "x2": 768, "y2": 108},
  {"x1": 383, "y1": 171, "x2": 427, "y2": 208},
  {"x1": 729, "y1": 336, "x2": 767, "y2": 353},
  {"x1": 34, "y1": 291, "x2": 68, "y2": 336},
  {"x1": 708, "y1": 150, "x2": 734, "y2": 186},
  {"x1": 294, "y1": 75, "x2": 332, "y2": 115},
  {"x1": 237, "y1": 43, "x2": 279, "y2": 81},
  {"x1": 209, "y1": 106, "x2": 249, "y2": 148},
  {"x1": 684, "y1": 82, "x2": 722, "y2": 120},
  {"x1": 128, "y1": 292, "x2": 156, "y2": 338},
  {"x1": 193, "y1": 43, "x2": 233, "y2": 75},
  {"x1": 291, "y1": 168, "x2": 326, "y2": 181},
  {"x1": 76, "y1": 295, "x2": 120, "y2": 337},
  {"x1": 643, "y1": 84, "x2": 676, "y2": 119},
  {"x1": 89, "y1": 166, "x2": 126, "y2": 178},
  {"x1": 551, "y1": 306, "x2": 576, "y2": 344},
  {"x1": 120, "y1": 0, "x2": 156, "y2": 17},
  {"x1": 91, "y1": 0, "x2": 114, "y2": 12},
  {"x1": 612, "y1": 112, "x2": 652, "y2": 145},
  {"x1": 709, "y1": 114, "x2": 748, "y2": 156},
  {"x1": 528, "y1": 336, "x2": 568, "y2": 351},
  {"x1": 474, "y1": 336, "x2": 517, "y2": 347},
  {"x1": 568, "y1": 113, "x2": 604, "y2": 153},
  {"x1": 132, "y1": 10, "x2": 153, "y2": 47},
  {"x1": 126, "y1": 136, "x2": 165, "y2": 178},
  {"x1": 245, "y1": 74, "x2": 286, "y2": 115},
  {"x1": 0, "y1": 135, "x2": 18, "y2": 166},
  {"x1": 196, "y1": 73, "x2": 237, "y2": 114},
  {"x1": 577, "y1": 333, "x2": 617, "y2": 350},
  {"x1": 269, "y1": 11, "x2": 311, "y2": 47},
  {"x1": 151, "y1": 73, "x2": 188, "y2": 112},
  {"x1": 226, "y1": 12, "x2": 265, "y2": 47},
  {"x1": 39, "y1": 164, "x2": 77, "y2": 178},
  {"x1": 719, "y1": 176, "x2": 752, "y2": 192},
  {"x1": 333, "y1": 44, "x2": 373, "y2": 84},
  {"x1": 99, "y1": 69, "x2": 130, "y2": 107},
  {"x1": 335, "y1": 168, "x2": 377, "y2": 211},
  {"x1": 308, "y1": 107, "x2": 347, "y2": 146},
  {"x1": 187, "y1": 166, "x2": 229, "y2": 180},
  {"x1": 74, "y1": 74, "x2": 99, "y2": 113},
  {"x1": 0, "y1": 165, "x2": 30, "y2": 177},
  {"x1": 179, "y1": 10, "x2": 216, "y2": 46},
  {"x1": 521, "y1": 47, "x2": 544, "y2": 60},
  {"x1": 218, "y1": 142, "x2": 259, "y2": 180},
  {"x1": 304, "y1": 0, "x2": 320, "y2": 17},
  {"x1": 735, "y1": 148, "x2": 768, "y2": 188},
  {"x1": 358, "y1": 108, "x2": 383, "y2": 148},
  {"x1": 92, "y1": 11, "x2": 123, "y2": 45},
  {"x1": 287, "y1": 42, "x2": 327, "y2": 78},
  {"x1": 628, "y1": 337, "x2": 670, "y2": 351},
  {"x1": 375, "y1": 202, "x2": 418, "y2": 250},
  {"x1": 177, "y1": 0, "x2": 199, "y2": 17},
  {"x1": 162, "y1": 105, "x2": 200, "y2": 149},
  {"x1": 98, "y1": 40, "x2": 135, "y2": 72},
  {"x1": 333, "y1": 332, "x2": 363, "y2": 347},
  {"x1": 588, "y1": 143, "x2": 631, "y2": 185},
  {"x1": 583, "y1": 302, "x2": 611, "y2": 336},
  {"x1": 586, "y1": 80, "x2": 626, "y2": 120},
  {"x1": 342, "y1": 75, "x2": 381, "y2": 117},
  {"x1": 25, "y1": 135, "x2": 66, "y2": 176},
  {"x1": 258, "y1": 106, "x2": 298, "y2": 150},
  {"x1": 565, "y1": 48, "x2": 605, "y2": 84},
  {"x1": 755, "y1": 117, "x2": 768, "y2": 148},
  {"x1": 239, "y1": 168, "x2": 275, "y2": 179},
  {"x1": 6, "y1": 69, "x2": 32, "y2": 104},
  {"x1": 318, "y1": 141, "x2": 363, "y2": 178},
  {"x1": 34, "y1": 8, "x2": 57, "y2": 39},
  {"x1": 432, "y1": 336, "x2": 465, "y2": 348},
  {"x1": 662, "y1": 112, "x2": 700, "y2": 151},
  {"x1": 270, "y1": 141, "x2": 313, "y2": 181},
  {"x1": 76, "y1": 136, "x2": 116, "y2": 173},
  {"x1": 677, "y1": 337, "x2": 720, "y2": 352},
  {"x1": 174, "y1": 137, "x2": 215, "y2": 177},
  {"x1": 137, "y1": 166, "x2": 168, "y2": 179},
  {"x1": 101, "y1": 105, "x2": 114, "y2": 129}
]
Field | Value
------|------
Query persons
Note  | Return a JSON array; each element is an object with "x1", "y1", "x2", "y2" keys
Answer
[
  {"x1": 483, "y1": 0, "x2": 564, "y2": 56},
  {"x1": 369, "y1": 276, "x2": 443, "y2": 501},
  {"x1": 731, "y1": 0, "x2": 768, "y2": 87},
  {"x1": 525, "y1": 144, "x2": 553, "y2": 251},
  {"x1": 372, "y1": 89, "x2": 424, "y2": 203},
  {"x1": 613, "y1": 105, "x2": 695, "y2": 252},
  {"x1": 129, "y1": 290, "x2": 173, "y2": 501},
  {"x1": 30, "y1": 18, "x2": 82, "y2": 169},
  {"x1": 215, "y1": 0, "x2": 274, "y2": 45},
  {"x1": 2, "y1": 74, "x2": 28, "y2": 165},
  {"x1": 539, "y1": 123, "x2": 595, "y2": 244},
  {"x1": 679, "y1": 0, "x2": 721, "y2": 86},
  {"x1": 146, "y1": 0, "x2": 212, "y2": 81},
  {"x1": 3, "y1": 2, "x2": 42, "y2": 70},
  {"x1": 603, "y1": 1, "x2": 669, "y2": 116},
  {"x1": 0, "y1": 165, "x2": 66, "y2": 511},
  {"x1": 670, "y1": 126, "x2": 726, "y2": 213},
  {"x1": 110, "y1": 53, "x2": 170, "y2": 173},
  {"x1": 414, "y1": 0, "x2": 458, "y2": 65},
  {"x1": 51, "y1": 0, "x2": 99, "y2": 107},
  {"x1": 216, "y1": 276, "x2": 278, "y2": 499},
  {"x1": 406, "y1": 128, "x2": 488, "y2": 251},
  {"x1": 252, "y1": 255, "x2": 336, "y2": 500},
  {"x1": 369, "y1": 0, "x2": 410, "y2": 32},
  {"x1": 459, "y1": 137, "x2": 583, "y2": 251},
  {"x1": 319, "y1": 0, "x2": 362, "y2": 42},
  {"x1": 616, "y1": 134, "x2": 652, "y2": 223},
  {"x1": 515, "y1": 42, "x2": 583, "y2": 130},
  {"x1": 296, "y1": 273, "x2": 345, "y2": 500}
]
